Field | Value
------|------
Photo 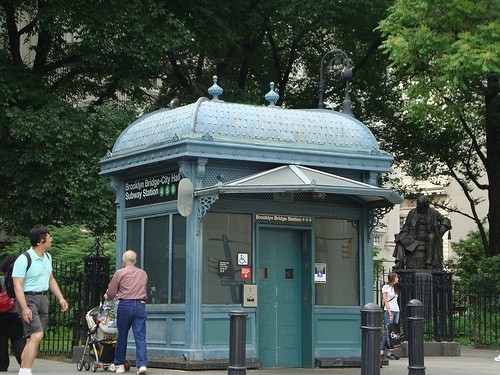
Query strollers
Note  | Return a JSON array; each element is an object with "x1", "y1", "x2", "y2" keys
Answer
[{"x1": 77, "y1": 296, "x2": 130, "y2": 373}]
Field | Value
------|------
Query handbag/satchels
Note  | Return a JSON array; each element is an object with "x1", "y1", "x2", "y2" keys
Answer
[{"x1": 0, "y1": 289, "x2": 15, "y2": 313}]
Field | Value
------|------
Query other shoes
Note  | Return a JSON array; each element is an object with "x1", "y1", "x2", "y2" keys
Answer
[
  {"x1": 380, "y1": 354, "x2": 390, "y2": 359},
  {"x1": 494, "y1": 354, "x2": 500, "y2": 362},
  {"x1": 386, "y1": 354, "x2": 400, "y2": 360},
  {"x1": 110, "y1": 364, "x2": 126, "y2": 373},
  {"x1": 137, "y1": 365, "x2": 147, "y2": 374}
]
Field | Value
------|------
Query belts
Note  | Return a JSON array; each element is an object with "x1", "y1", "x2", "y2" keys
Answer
[
  {"x1": 25, "y1": 291, "x2": 47, "y2": 295},
  {"x1": 121, "y1": 299, "x2": 146, "y2": 302}
]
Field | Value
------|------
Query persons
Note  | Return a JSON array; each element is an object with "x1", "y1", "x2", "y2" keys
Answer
[
  {"x1": 0, "y1": 254, "x2": 27, "y2": 372},
  {"x1": 11, "y1": 227, "x2": 68, "y2": 375},
  {"x1": 381, "y1": 273, "x2": 400, "y2": 358},
  {"x1": 392, "y1": 195, "x2": 451, "y2": 274},
  {"x1": 103, "y1": 250, "x2": 149, "y2": 373}
]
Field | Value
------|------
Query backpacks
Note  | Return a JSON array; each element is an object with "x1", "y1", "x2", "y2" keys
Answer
[{"x1": 4, "y1": 251, "x2": 32, "y2": 298}]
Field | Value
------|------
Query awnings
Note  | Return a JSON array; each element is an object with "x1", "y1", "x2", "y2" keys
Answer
[{"x1": 194, "y1": 166, "x2": 405, "y2": 239}]
